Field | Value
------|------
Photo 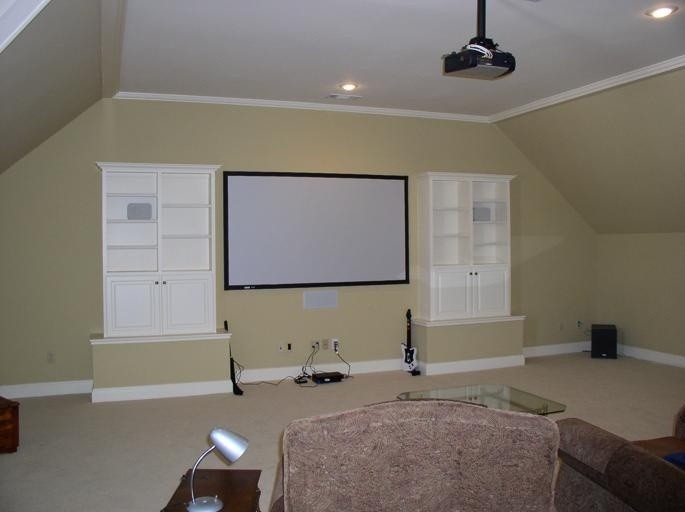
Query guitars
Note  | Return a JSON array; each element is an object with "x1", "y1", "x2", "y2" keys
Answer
[{"x1": 400, "y1": 310, "x2": 418, "y2": 372}]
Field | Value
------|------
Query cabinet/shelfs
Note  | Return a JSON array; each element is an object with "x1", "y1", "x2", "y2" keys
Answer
[
  {"x1": 95, "y1": 162, "x2": 223, "y2": 339},
  {"x1": 416, "y1": 171, "x2": 517, "y2": 321}
]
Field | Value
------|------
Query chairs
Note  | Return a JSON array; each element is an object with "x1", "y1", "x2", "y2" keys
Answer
[
  {"x1": 269, "y1": 398, "x2": 559, "y2": 512},
  {"x1": 555, "y1": 404, "x2": 685, "y2": 512}
]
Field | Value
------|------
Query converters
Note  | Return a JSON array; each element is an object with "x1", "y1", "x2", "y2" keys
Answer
[{"x1": 295, "y1": 378, "x2": 307, "y2": 383}]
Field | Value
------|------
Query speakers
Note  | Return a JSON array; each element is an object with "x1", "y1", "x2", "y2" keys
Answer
[{"x1": 591, "y1": 324, "x2": 617, "y2": 359}]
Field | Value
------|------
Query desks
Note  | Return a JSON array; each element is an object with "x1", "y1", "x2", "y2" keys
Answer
[{"x1": 162, "y1": 469, "x2": 262, "y2": 512}]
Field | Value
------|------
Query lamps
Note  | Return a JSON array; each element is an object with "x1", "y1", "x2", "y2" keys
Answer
[{"x1": 185, "y1": 427, "x2": 250, "y2": 512}]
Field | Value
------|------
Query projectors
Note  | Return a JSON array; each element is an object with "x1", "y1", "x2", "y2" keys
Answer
[{"x1": 444, "y1": 49, "x2": 516, "y2": 80}]
